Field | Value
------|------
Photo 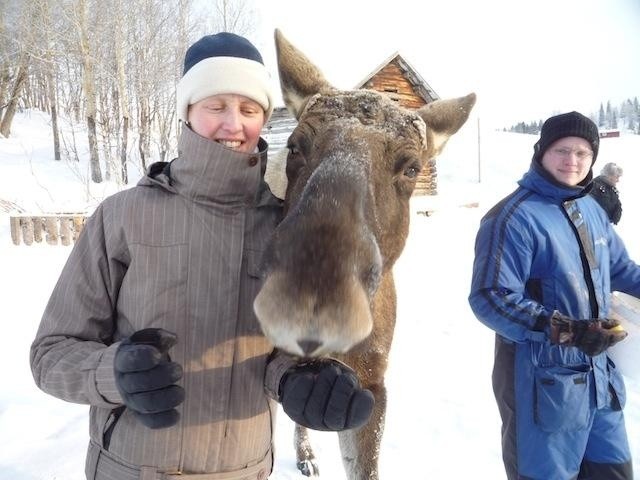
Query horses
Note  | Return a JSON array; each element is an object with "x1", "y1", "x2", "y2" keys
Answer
[{"x1": 253, "y1": 27, "x2": 476, "y2": 480}]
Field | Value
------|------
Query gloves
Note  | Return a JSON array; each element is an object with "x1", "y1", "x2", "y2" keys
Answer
[
  {"x1": 280, "y1": 362, "x2": 376, "y2": 432},
  {"x1": 113, "y1": 328, "x2": 186, "y2": 430},
  {"x1": 548, "y1": 311, "x2": 628, "y2": 357}
]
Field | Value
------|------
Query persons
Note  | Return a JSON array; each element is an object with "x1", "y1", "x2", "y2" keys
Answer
[
  {"x1": 467, "y1": 110, "x2": 640, "y2": 480},
  {"x1": 28, "y1": 31, "x2": 375, "y2": 480},
  {"x1": 588, "y1": 162, "x2": 623, "y2": 226}
]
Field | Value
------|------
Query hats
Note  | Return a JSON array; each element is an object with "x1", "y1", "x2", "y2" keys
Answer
[
  {"x1": 539, "y1": 111, "x2": 599, "y2": 164},
  {"x1": 177, "y1": 32, "x2": 273, "y2": 127}
]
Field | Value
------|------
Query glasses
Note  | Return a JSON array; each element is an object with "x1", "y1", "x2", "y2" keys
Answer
[{"x1": 550, "y1": 144, "x2": 593, "y2": 159}]
